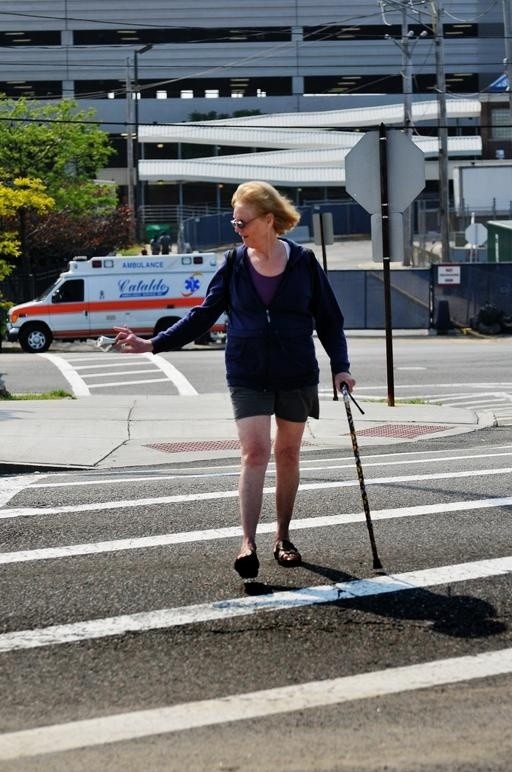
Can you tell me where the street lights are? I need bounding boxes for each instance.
[132,41,155,245]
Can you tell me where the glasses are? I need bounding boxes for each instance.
[230,215,260,229]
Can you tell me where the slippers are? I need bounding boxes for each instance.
[235,552,259,578]
[273,540,301,567]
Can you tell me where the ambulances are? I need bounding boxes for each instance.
[6,251,228,354]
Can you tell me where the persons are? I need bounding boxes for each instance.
[109,179,359,579]
[158,231,172,255]
[149,234,160,255]
